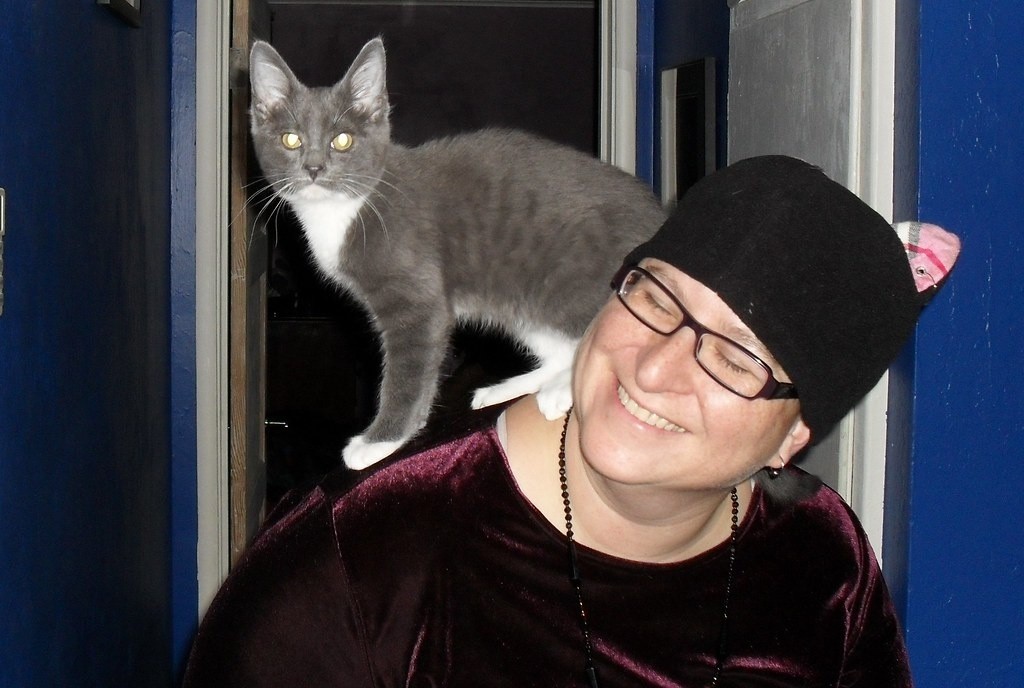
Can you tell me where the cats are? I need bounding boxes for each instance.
[249,37,667,470]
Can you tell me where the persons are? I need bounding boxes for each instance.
[178,153,922,688]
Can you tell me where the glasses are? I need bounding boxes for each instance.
[615,265,800,400]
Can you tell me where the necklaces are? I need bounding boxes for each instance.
[559,403,741,688]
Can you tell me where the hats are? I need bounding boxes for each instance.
[609,154,925,449]
